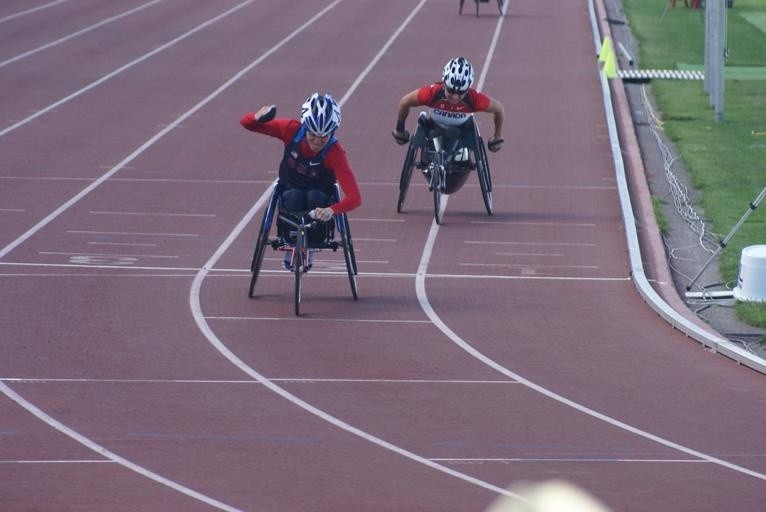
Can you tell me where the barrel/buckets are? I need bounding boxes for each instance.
[726,245,766,304]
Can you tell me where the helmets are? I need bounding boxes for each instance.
[300,91,343,137]
[441,56,475,93]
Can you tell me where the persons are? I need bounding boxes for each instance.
[393,57,505,194]
[240,92,362,272]
